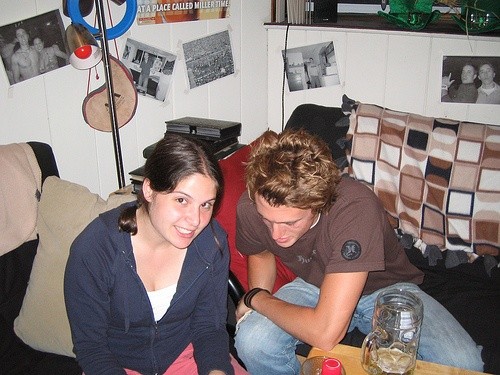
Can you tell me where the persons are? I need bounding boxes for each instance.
[307,58,321,88]
[64,133,251,375]
[2,27,69,83]
[441,61,500,105]
[234,129,484,375]
[124,46,152,92]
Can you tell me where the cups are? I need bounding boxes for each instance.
[360,289,424,375]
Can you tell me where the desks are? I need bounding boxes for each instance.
[301,343,495,375]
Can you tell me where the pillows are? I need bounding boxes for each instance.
[13,176,137,358]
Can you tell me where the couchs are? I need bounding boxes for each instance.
[211,94,500,375]
[0,142,59,375]
